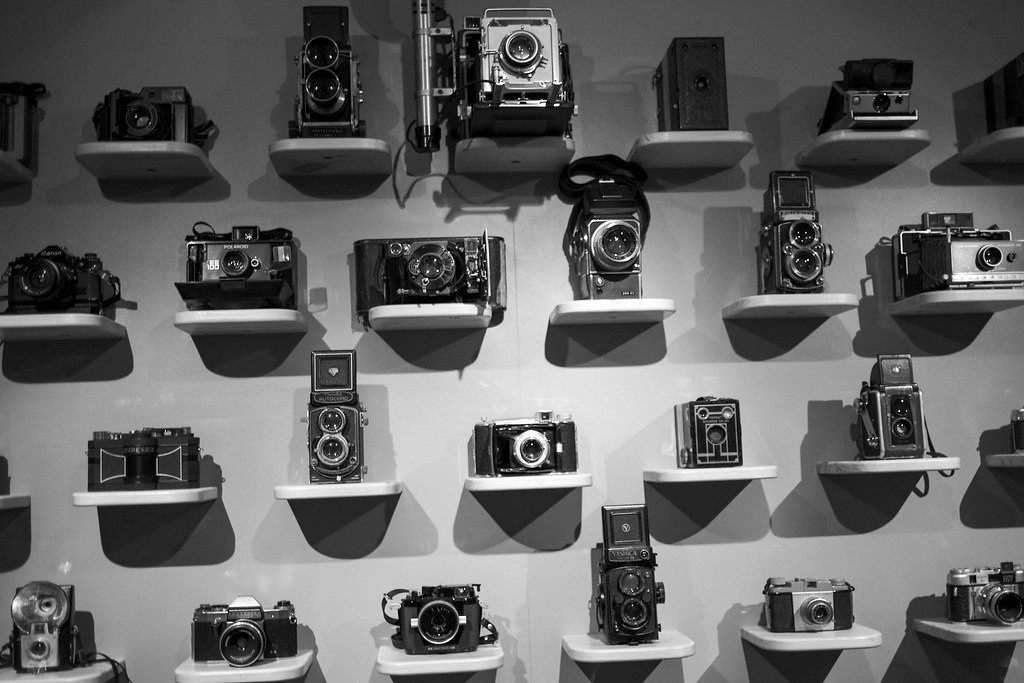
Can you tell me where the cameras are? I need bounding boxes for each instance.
[397,585,483,656]
[596,503,666,647]
[10,580,80,672]
[946,561,1024,625]
[0,0,1024,493]
[189,596,298,667]
[763,577,854,633]
